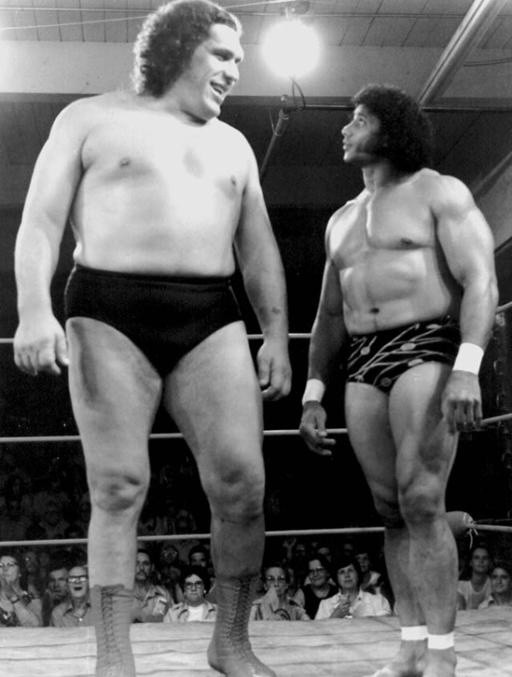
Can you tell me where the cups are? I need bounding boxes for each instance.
[338,593,353,617]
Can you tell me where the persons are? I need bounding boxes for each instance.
[12,1,293,677]
[298,83,500,677]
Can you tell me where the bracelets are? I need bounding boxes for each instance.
[452,341,484,376]
[300,378,328,406]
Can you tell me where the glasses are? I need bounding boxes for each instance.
[0,561,20,569]
[66,575,87,582]
[266,575,285,581]
[184,581,205,589]
